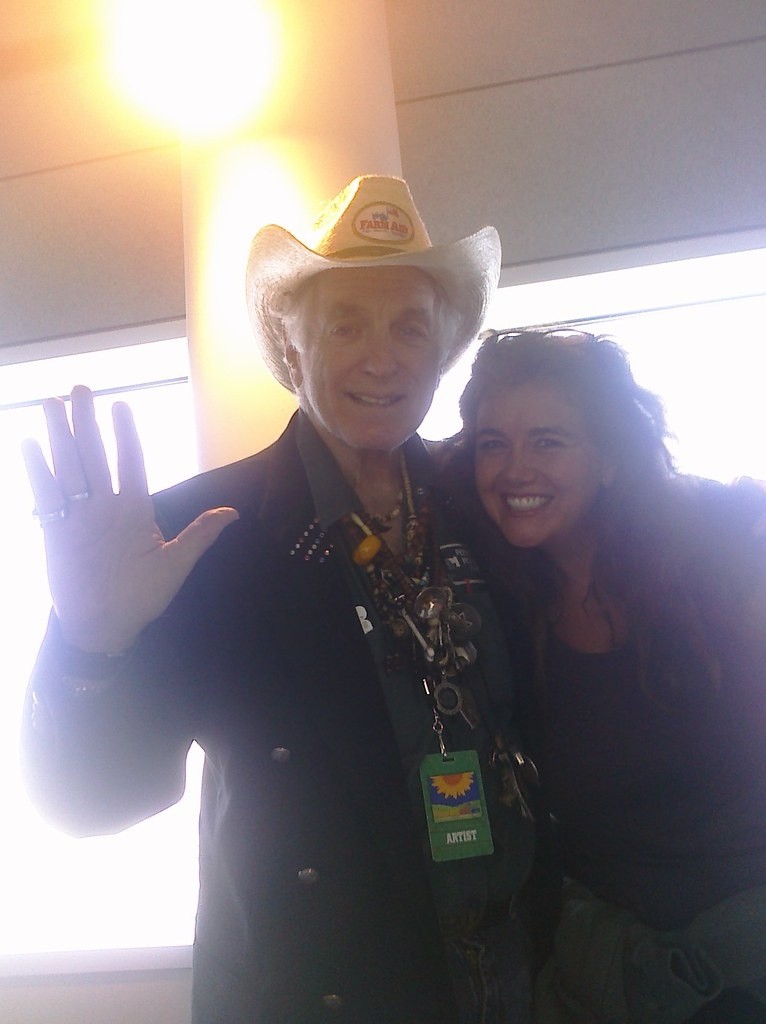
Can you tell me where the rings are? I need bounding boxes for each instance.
[63,490,89,503]
[33,508,68,526]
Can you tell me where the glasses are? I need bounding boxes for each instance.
[489,326,595,352]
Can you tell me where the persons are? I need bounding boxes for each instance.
[435,329,764,1024]
[22,176,536,1024]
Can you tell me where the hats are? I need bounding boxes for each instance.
[252,175,500,395]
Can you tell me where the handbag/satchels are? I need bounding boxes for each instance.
[533,872,766,1024]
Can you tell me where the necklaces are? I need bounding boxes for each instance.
[343,446,481,729]
